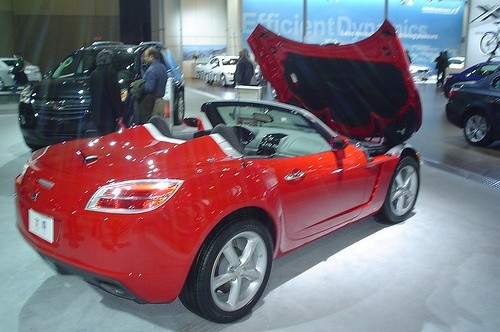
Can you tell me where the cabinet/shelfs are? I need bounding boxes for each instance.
[234,85,272,126]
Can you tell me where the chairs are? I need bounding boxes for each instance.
[210,123,244,155]
[147,115,173,138]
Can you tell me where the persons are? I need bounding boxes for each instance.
[228,48,255,120]
[89,49,123,136]
[9,52,29,93]
[130,46,168,126]
[434,51,451,85]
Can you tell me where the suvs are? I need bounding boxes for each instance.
[17,40,186,151]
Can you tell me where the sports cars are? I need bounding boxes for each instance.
[16,19,422,328]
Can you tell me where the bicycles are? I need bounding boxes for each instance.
[478,21,500,55]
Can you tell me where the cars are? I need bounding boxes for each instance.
[0,58,42,91]
[444,69,500,146]
[441,60,500,97]
[203,56,242,87]
